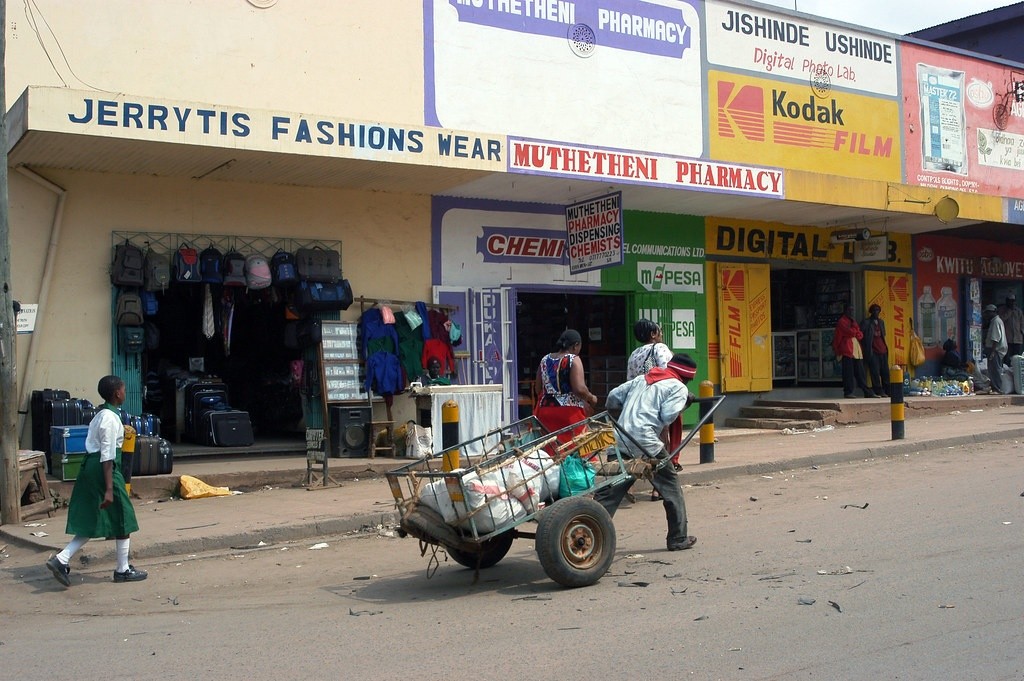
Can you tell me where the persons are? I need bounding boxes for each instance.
[46,376,148,586]
[537,327,598,463]
[605,353,697,550]
[997,294,1024,365]
[858,305,892,395]
[940,339,988,393]
[983,304,1009,395]
[834,303,880,397]
[628,319,683,502]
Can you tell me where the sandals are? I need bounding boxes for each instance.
[651,488,663,500]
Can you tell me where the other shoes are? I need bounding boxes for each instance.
[872,394,881,398]
[989,389,1000,395]
[674,464,683,472]
[668,536,697,551]
[844,394,856,398]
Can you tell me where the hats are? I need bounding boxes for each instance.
[427,357,441,368]
[983,304,996,313]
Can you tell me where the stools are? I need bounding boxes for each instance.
[366,420,397,457]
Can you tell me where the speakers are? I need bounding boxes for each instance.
[330,405,372,458]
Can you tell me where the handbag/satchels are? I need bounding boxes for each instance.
[296,276,354,310]
[405,424,432,458]
[643,343,657,374]
[559,451,597,498]
[296,246,340,282]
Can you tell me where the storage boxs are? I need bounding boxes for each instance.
[51,452,86,481]
[50,425,89,454]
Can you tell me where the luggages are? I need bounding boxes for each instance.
[183,378,256,445]
[31,389,172,482]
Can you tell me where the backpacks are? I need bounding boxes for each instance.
[112,238,298,353]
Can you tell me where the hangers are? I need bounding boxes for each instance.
[434,331,440,340]
[429,304,438,312]
[377,340,387,353]
[370,299,380,309]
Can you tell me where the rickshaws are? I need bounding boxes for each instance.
[386,394,727,586]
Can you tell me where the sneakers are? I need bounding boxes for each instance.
[114,565,147,582]
[46,555,71,587]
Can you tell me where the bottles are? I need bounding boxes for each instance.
[936,286,958,351]
[903,372,974,397]
[917,286,937,349]
